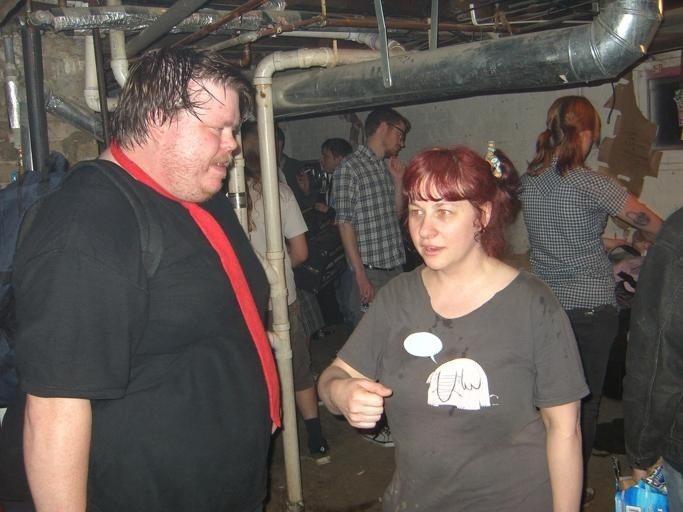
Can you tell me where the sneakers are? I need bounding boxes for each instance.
[582,488,594,506]
[359,427,395,448]
[307,447,331,465]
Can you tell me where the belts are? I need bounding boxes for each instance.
[364,264,391,270]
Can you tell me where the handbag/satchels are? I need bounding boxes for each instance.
[309,323,355,381]
[287,203,346,295]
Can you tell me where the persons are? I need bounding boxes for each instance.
[316,145,589,511]
[225,106,413,464]
[621,206,683,511]
[516,95,665,505]
[13,47,283,512]
